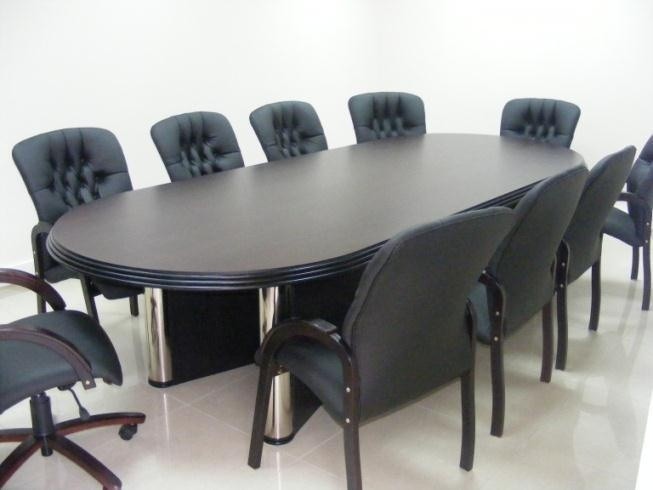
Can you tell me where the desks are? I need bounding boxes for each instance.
[44,133,584,387]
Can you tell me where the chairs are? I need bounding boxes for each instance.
[11,127,149,326]
[501,99,580,148]
[250,100,327,161]
[247,207,517,488]
[150,112,245,182]
[562,144,637,370]
[607,134,652,312]
[0,267,146,489]
[474,165,590,436]
[348,91,427,143]
